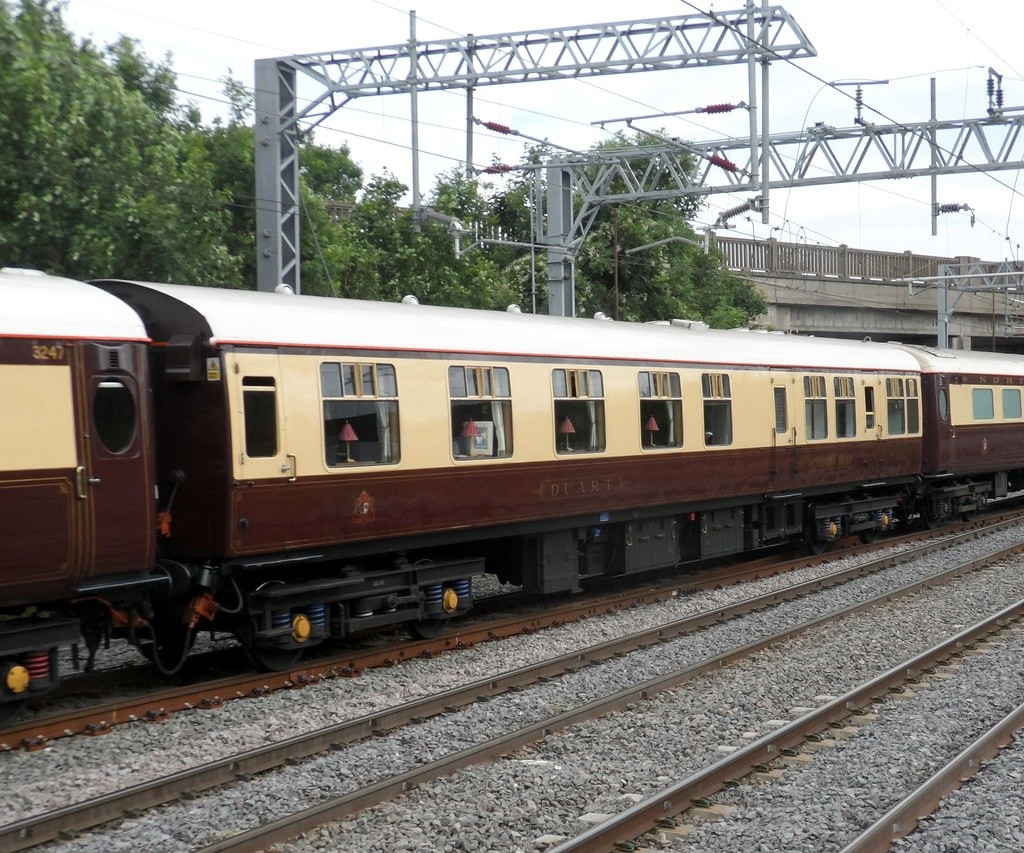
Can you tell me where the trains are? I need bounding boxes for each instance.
[1,261,1024,717]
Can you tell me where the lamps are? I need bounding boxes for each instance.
[642,414,661,446]
[338,419,358,464]
[559,415,576,453]
[461,418,480,458]
